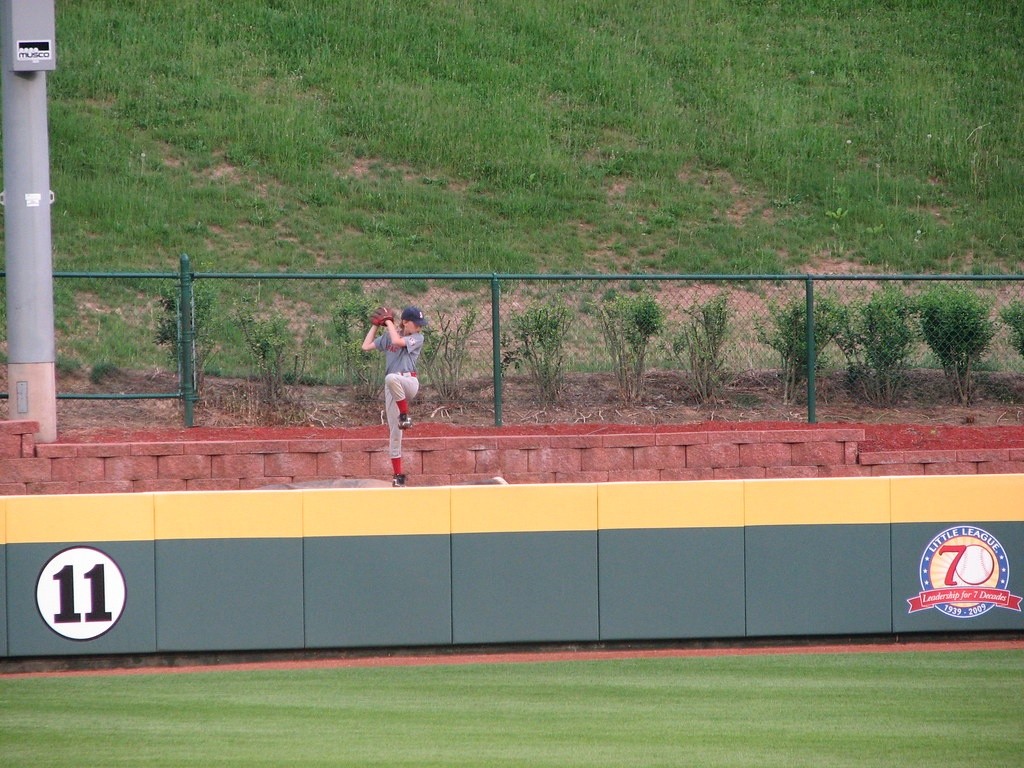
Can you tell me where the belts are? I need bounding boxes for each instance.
[401,372,416,377]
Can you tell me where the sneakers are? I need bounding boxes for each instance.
[398,413,412,429]
[392,473,406,487]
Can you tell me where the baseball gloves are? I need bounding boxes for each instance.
[369,306,395,326]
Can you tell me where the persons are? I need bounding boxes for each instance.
[361,306,429,488]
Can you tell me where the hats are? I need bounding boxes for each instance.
[401,306,427,326]
